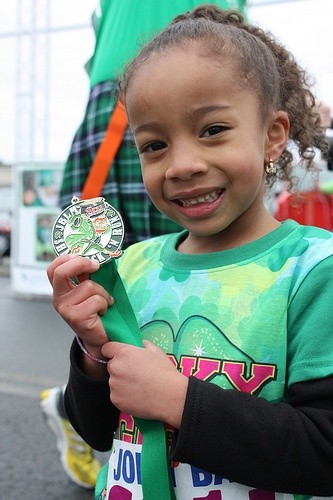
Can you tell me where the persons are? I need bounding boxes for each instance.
[46,6,333,500]
[37,0,248,489]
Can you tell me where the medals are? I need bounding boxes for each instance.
[51,194,125,267]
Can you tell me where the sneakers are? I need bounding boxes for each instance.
[37,382,101,490]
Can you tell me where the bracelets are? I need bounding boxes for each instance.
[75,332,109,367]
